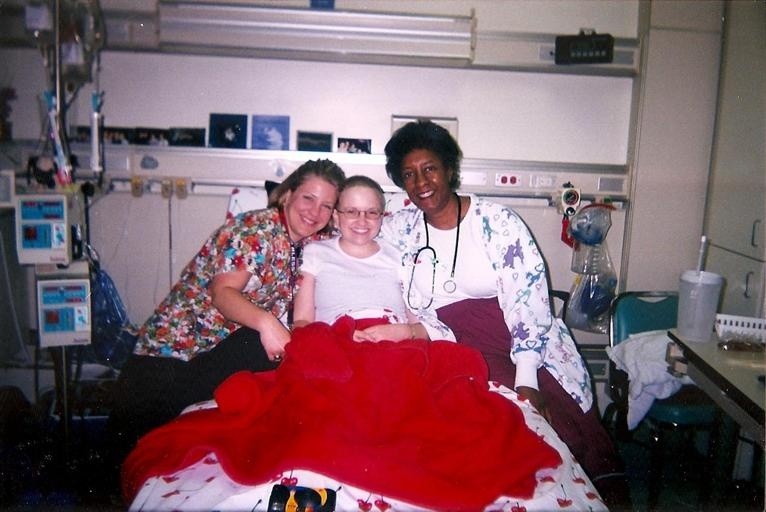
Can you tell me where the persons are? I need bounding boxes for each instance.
[378,119,595,416]
[116,158,347,429]
[292,176,456,343]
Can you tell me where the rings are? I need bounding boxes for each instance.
[275,356,280,359]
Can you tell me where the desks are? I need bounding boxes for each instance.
[665,324,765,509]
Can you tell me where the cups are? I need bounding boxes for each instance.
[678,268,724,344]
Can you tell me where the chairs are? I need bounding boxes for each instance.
[603,291,716,454]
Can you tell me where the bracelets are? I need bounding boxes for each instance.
[409,323,416,340]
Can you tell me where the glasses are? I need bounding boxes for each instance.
[336,206,382,220]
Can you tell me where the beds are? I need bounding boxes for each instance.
[134,186,609,512]
